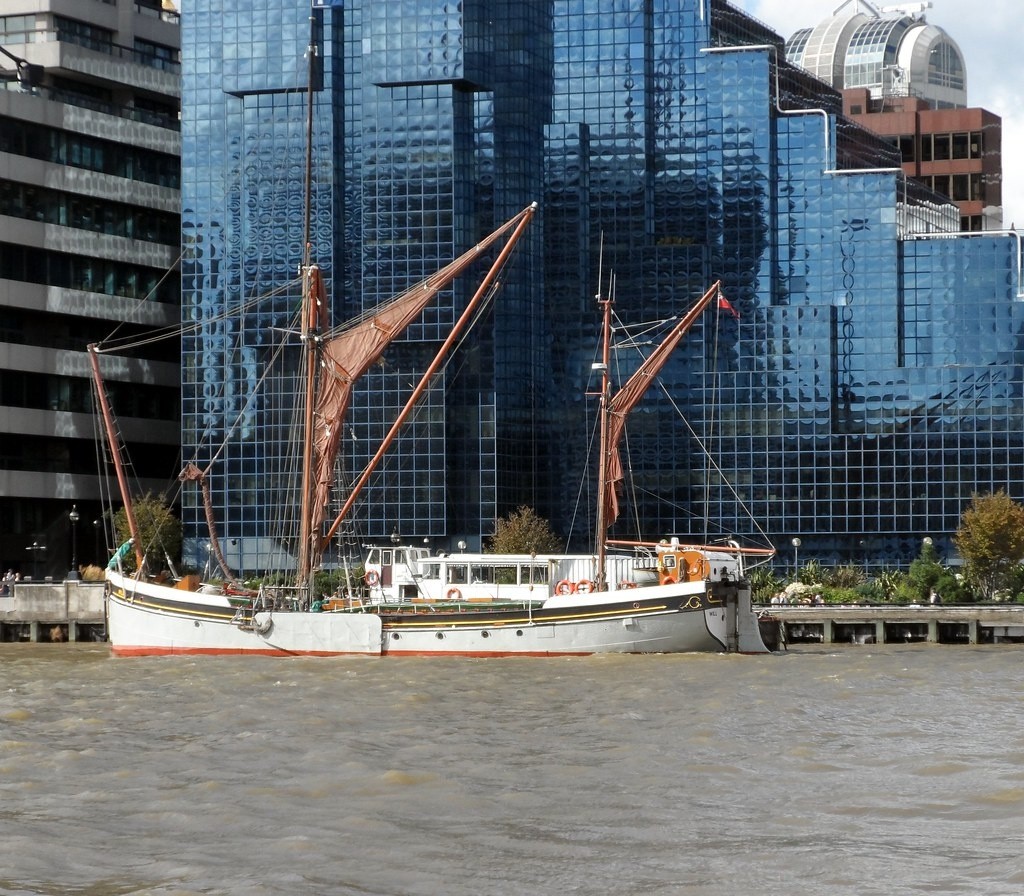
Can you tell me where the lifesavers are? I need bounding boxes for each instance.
[662,576,676,585]
[555,579,574,596]
[618,580,636,589]
[364,569,379,586]
[574,579,594,594]
[447,588,461,599]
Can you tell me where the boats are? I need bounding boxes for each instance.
[85,13,776,658]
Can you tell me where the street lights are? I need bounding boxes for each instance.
[793,538,801,604]
[26,542,48,580]
[67,505,79,579]
[458,541,466,554]
[92,520,101,567]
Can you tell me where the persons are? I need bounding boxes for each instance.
[770,590,825,606]
[0,568,22,597]
[929,586,944,607]
